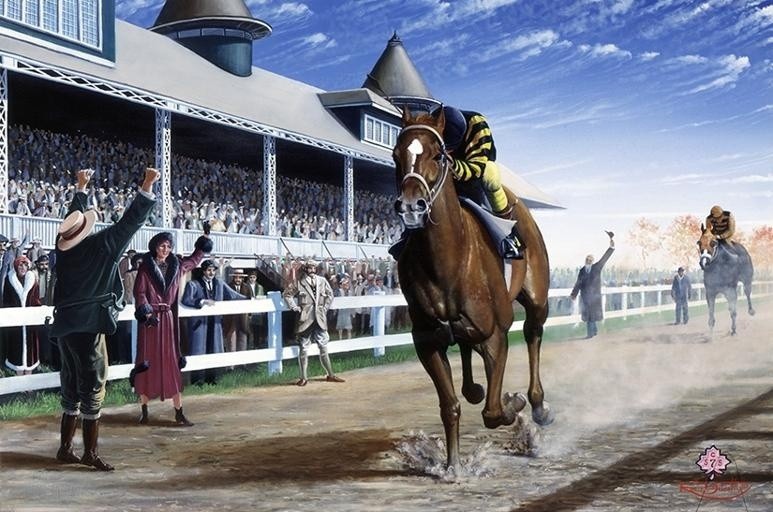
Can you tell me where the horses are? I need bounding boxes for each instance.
[696,222,755,338]
[391,102,550,479]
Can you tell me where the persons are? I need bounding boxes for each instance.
[670,269,694,324]
[569,232,615,336]
[706,206,737,250]
[435,107,521,256]
[0,124,405,470]
